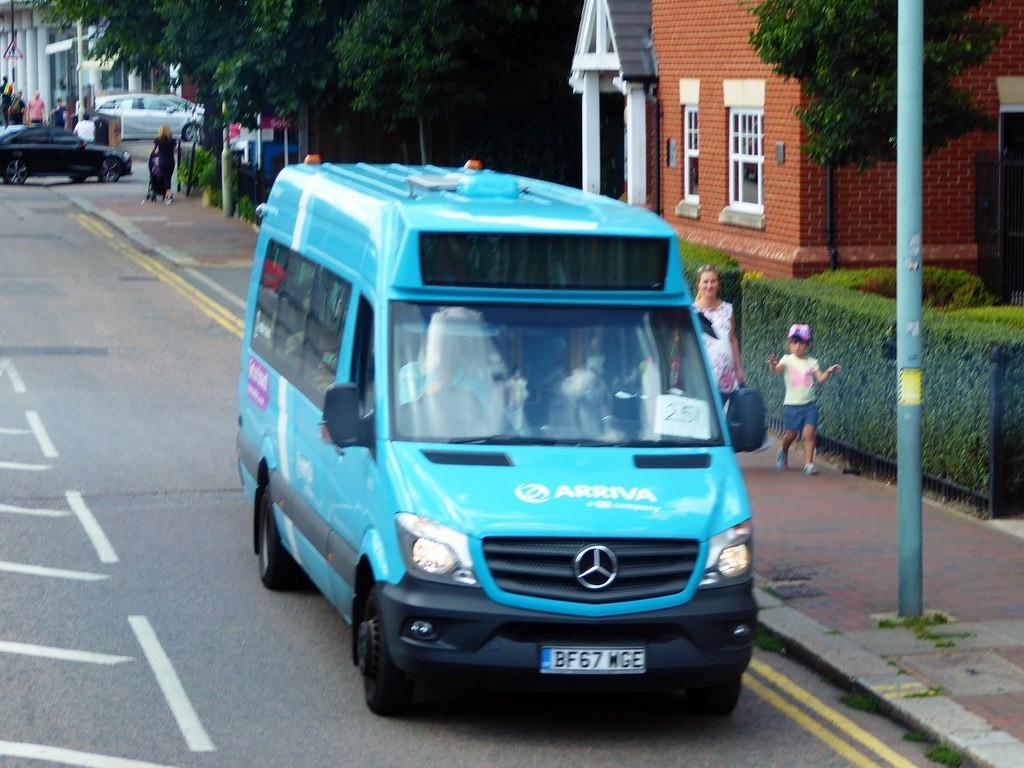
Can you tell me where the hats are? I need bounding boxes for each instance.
[787,323,812,343]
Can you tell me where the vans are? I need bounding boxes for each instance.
[238,154,764,717]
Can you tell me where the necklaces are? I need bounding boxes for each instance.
[705,298,717,308]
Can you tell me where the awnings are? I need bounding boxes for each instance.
[46,33,105,55]
[83,52,120,71]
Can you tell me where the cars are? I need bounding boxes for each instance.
[0,125,134,182]
[95,94,205,142]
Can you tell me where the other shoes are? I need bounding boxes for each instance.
[802,463,820,475]
[165,196,173,206]
[776,449,788,471]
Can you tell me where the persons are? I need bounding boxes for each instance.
[691,264,745,405]
[400,333,526,435]
[25,92,47,124]
[229,122,241,138]
[50,99,67,128]
[73,113,95,144]
[150,125,177,205]
[0,77,12,125]
[8,92,25,125]
[767,324,842,475]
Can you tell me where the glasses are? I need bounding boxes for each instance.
[697,265,715,273]
